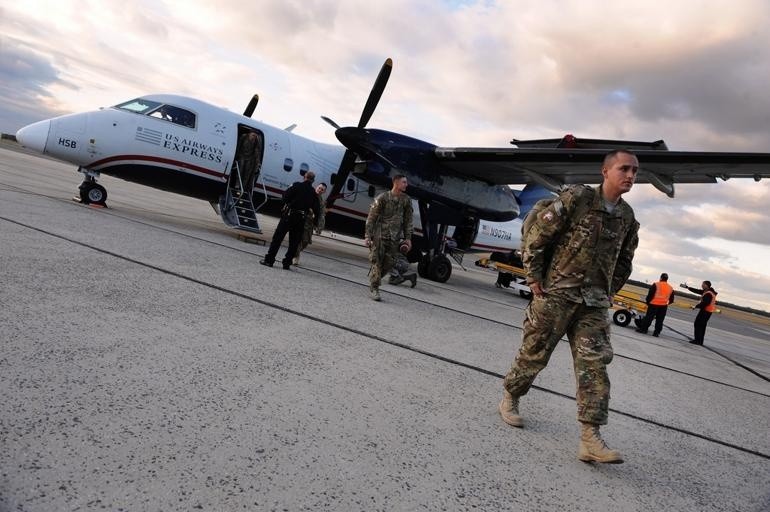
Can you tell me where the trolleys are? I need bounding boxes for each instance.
[471,252,648,330]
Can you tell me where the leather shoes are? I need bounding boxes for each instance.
[262,257,275,266]
[282,260,294,269]
[651,334,659,337]
[690,339,701,343]
[636,329,647,334]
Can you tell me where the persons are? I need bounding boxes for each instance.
[388,226,417,288]
[635,273,674,337]
[292,182,328,265]
[235,129,262,188]
[259,171,321,270]
[499,149,640,464]
[680,280,721,346]
[364,173,413,299]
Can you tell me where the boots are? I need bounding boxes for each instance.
[368,285,383,302]
[410,272,417,288]
[292,255,299,265]
[497,391,523,428]
[578,420,623,463]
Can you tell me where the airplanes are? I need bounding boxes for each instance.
[14,57,770,283]
